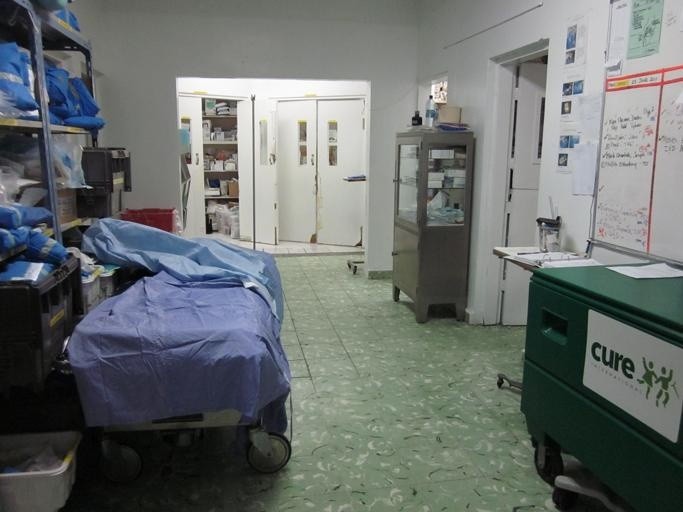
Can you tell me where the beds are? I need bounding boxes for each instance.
[48,235,293,474]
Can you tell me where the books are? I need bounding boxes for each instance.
[498,246,603,270]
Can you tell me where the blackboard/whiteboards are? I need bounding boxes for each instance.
[588,64,683,267]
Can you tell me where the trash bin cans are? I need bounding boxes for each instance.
[121,207,178,234]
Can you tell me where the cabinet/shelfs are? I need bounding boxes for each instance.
[179,94,253,242]
[0,1,99,267]
[392,131,474,324]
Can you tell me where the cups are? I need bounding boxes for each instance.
[537,220,561,251]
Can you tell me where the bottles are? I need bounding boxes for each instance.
[453,203,459,222]
[411,111,421,126]
[424,95,435,127]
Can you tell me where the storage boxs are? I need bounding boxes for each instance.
[54,130,91,191]
[56,188,79,224]
[1,432,85,512]
[2,251,120,399]
